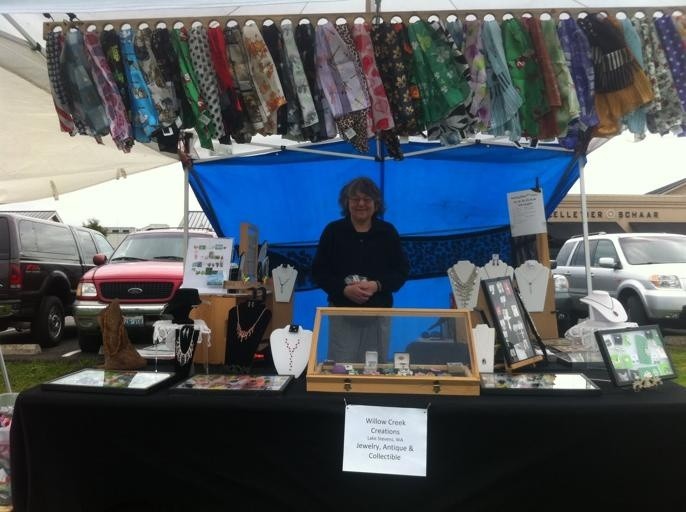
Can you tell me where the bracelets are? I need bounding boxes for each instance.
[376,280,381,293]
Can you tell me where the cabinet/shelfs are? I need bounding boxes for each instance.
[186,293,300,367]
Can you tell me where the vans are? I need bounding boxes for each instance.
[1,213,115,347]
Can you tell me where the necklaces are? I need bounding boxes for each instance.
[284,336,300,372]
[484,262,509,281]
[231,305,269,342]
[102,306,126,357]
[175,326,195,365]
[450,266,479,309]
[519,269,544,295]
[276,272,293,296]
[583,296,620,318]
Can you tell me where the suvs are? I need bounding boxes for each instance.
[75,230,216,352]
[557,233,686,327]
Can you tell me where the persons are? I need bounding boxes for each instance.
[311,175,411,363]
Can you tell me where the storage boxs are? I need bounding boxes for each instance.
[304,305,482,395]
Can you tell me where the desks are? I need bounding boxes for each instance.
[9,370,686,511]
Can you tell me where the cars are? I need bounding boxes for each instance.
[550,258,569,335]
